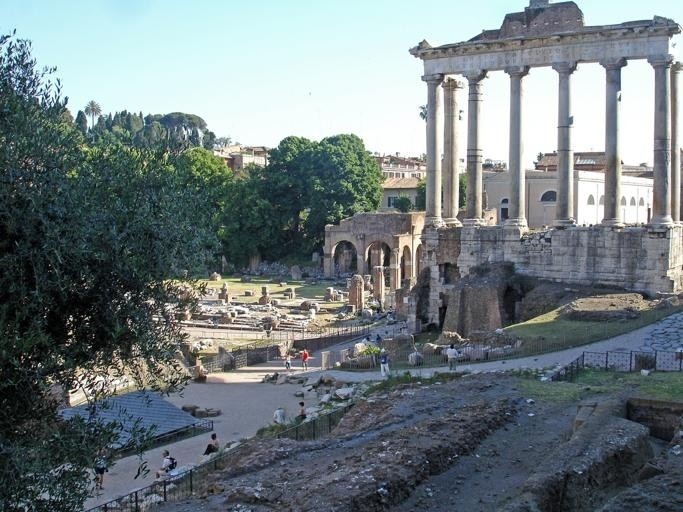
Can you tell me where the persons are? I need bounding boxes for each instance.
[201,432,220,456]
[301,348,309,371]
[446,342,458,371]
[93,444,106,491]
[378,349,391,377]
[154,449,172,479]
[271,406,285,426]
[282,351,296,371]
[360,330,390,346]
[295,401,307,422]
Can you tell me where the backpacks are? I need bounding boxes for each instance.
[167,457,177,469]
